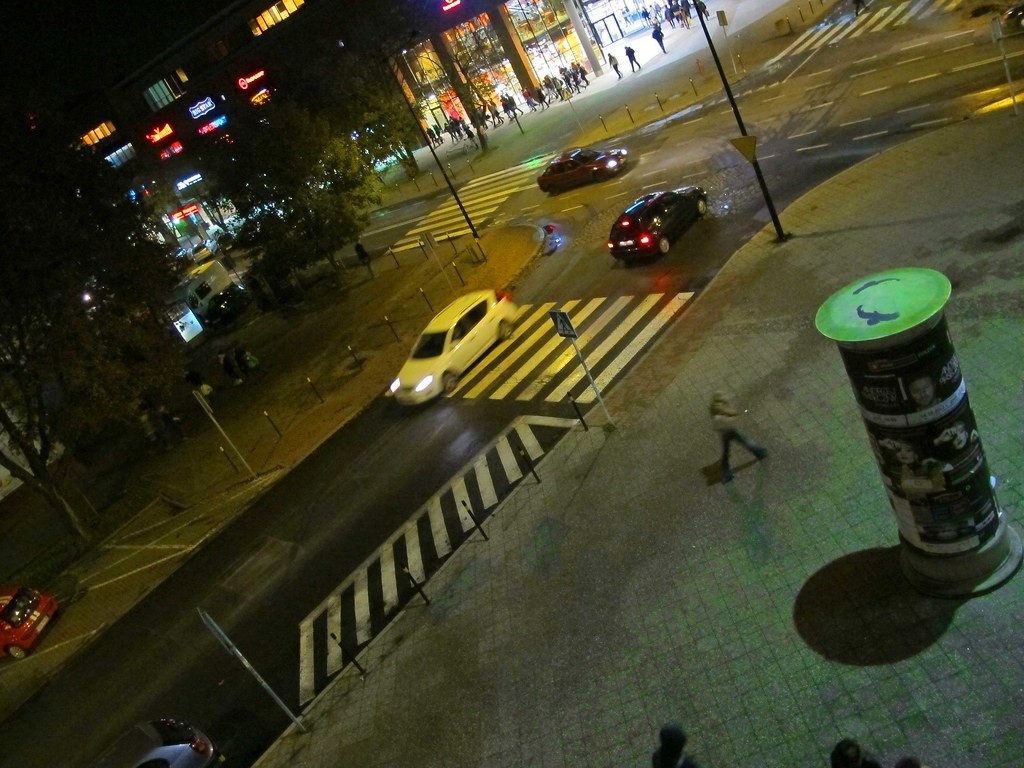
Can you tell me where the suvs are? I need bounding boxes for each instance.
[609,184,709,264]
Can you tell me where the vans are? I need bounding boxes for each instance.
[171,260,233,328]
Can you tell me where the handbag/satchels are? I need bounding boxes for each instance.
[712,415,737,433]
[494,109,500,117]
[583,68,589,75]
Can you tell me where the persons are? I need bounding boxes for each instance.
[651,721,699,768]
[707,390,768,485]
[640,0,709,30]
[652,27,666,54]
[607,53,623,80]
[624,47,641,72]
[830,737,921,767]
[426,61,590,149]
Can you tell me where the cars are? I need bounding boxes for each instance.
[386,287,515,406]
[1,583,58,661]
[538,143,628,196]
[194,237,221,265]
[173,246,194,262]
[208,282,252,322]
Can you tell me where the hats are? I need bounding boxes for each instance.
[713,390,725,401]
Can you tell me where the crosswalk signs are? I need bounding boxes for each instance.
[547,311,577,340]
